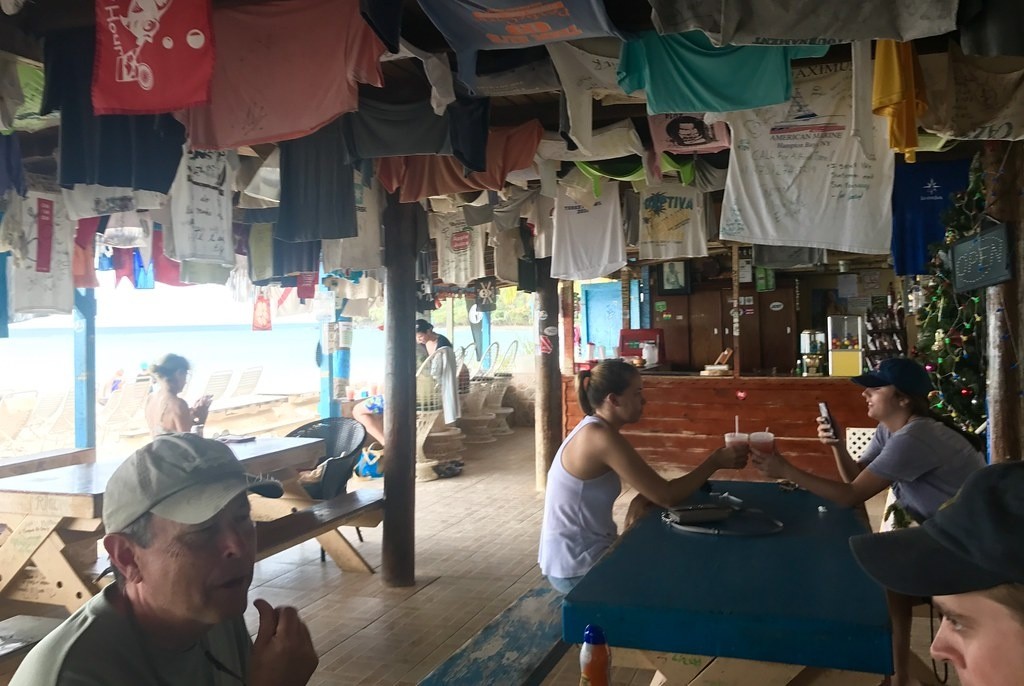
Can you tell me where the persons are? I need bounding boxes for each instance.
[749,357,988,686]
[144,355,213,437]
[415,318,453,361]
[537,359,751,597]
[849,460,1024,686]
[9,431,320,686]
[352,367,385,447]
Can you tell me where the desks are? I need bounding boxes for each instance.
[562,480,894,685]
[0,435,375,615]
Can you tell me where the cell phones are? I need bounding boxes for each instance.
[818,400,838,439]
[204,393,214,399]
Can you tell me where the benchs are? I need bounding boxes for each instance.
[255,489,382,563]
[416,576,572,686]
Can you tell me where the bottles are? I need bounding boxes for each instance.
[864,281,906,372]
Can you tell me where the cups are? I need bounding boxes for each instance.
[750,431,775,453]
[723,432,749,447]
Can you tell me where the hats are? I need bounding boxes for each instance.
[850,461,1024,596]
[102,432,284,532]
[850,358,933,396]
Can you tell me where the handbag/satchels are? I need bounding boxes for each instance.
[352,442,384,481]
[662,492,783,535]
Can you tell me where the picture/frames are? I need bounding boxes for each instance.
[656,260,692,295]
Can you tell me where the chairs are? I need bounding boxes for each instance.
[204,368,232,399]
[0,390,34,454]
[365,339,519,482]
[107,375,153,442]
[46,377,74,449]
[235,368,262,398]
[283,417,365,563]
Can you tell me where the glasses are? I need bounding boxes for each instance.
[416,324,421,329]
